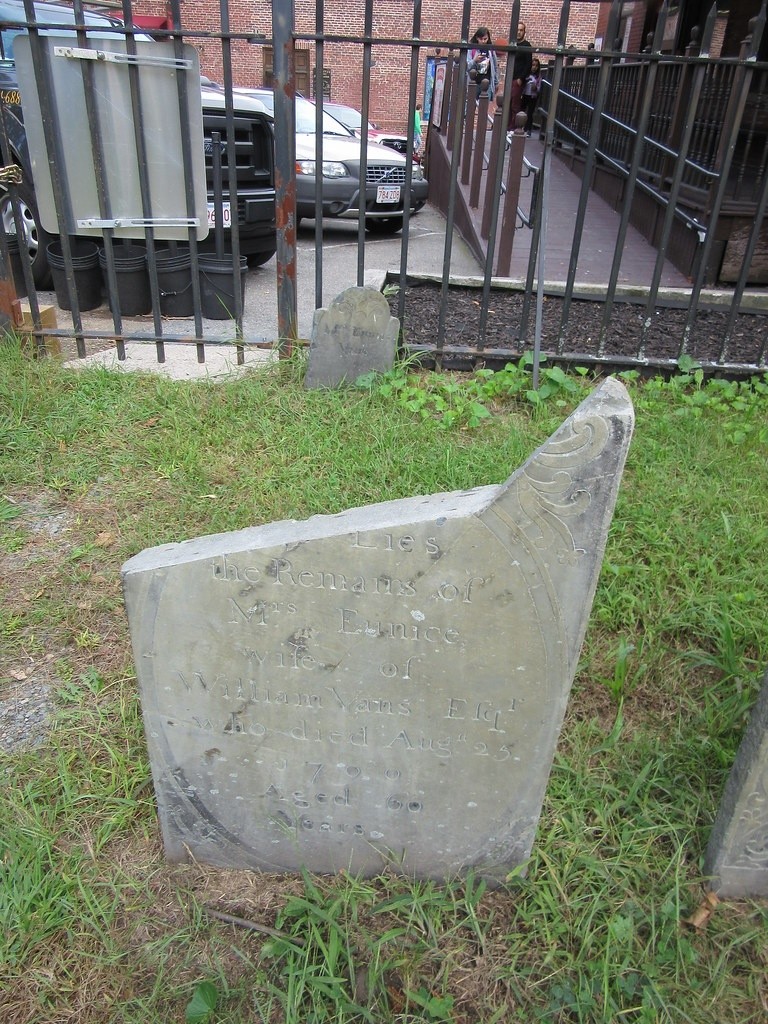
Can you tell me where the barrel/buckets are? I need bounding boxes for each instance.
[145,247,195,316]
[46,239,102,311]
[5,233,29,299]
[198,253,248,320]
[99,244,152,317]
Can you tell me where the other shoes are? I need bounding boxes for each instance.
[526,130,531,136]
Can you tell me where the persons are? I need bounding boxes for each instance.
[504,21,542,138]
[467,27,500,103]
[413,104,424,154]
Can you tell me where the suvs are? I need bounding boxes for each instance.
[217,85,430,237]
[1,0,279,293]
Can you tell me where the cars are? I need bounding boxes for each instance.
[306,97,420,167]
